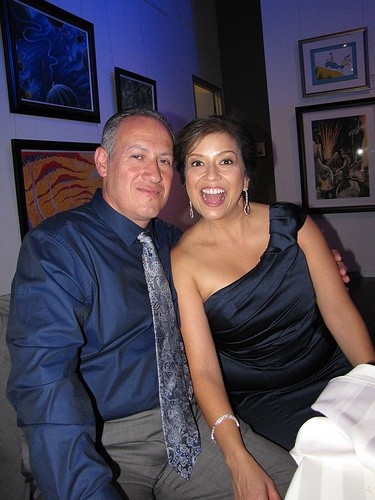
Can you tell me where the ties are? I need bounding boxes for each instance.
[138,231,203,479]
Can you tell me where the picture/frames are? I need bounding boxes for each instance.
[0,0,101,123]
[297,26,371,98]
[295,97,375,214]
[11,138,102,243]
[114,66,158,116]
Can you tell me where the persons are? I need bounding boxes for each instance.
[169,114,375,500]
[5,107,350,499]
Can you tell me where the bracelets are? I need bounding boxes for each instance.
[210,414,240,444]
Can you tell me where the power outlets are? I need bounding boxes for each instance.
[255,142,265,156]
